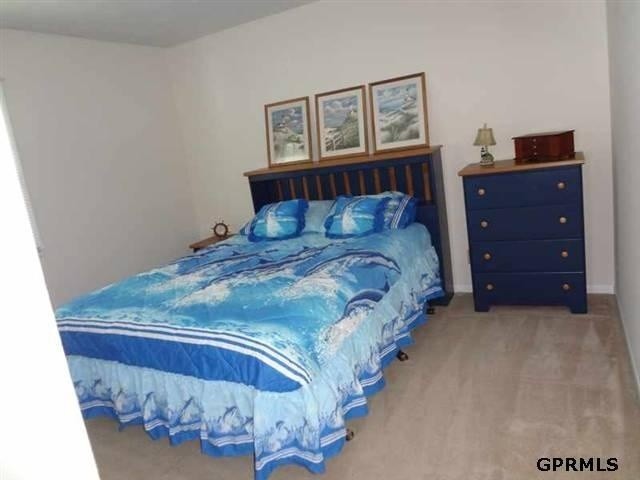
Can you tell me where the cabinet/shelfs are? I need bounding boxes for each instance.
[457,151,587,315]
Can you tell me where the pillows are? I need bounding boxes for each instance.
[321,189,420,239]
[240,199,335,243]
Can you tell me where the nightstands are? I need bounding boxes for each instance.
[188,235,229,252]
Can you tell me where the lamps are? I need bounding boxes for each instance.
[472,123,498,166]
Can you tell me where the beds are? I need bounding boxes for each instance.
[54,145,454,480]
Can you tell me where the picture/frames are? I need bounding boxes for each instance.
[368,72,429,155]
[264,95,312,169]
[314,85,369,162]
